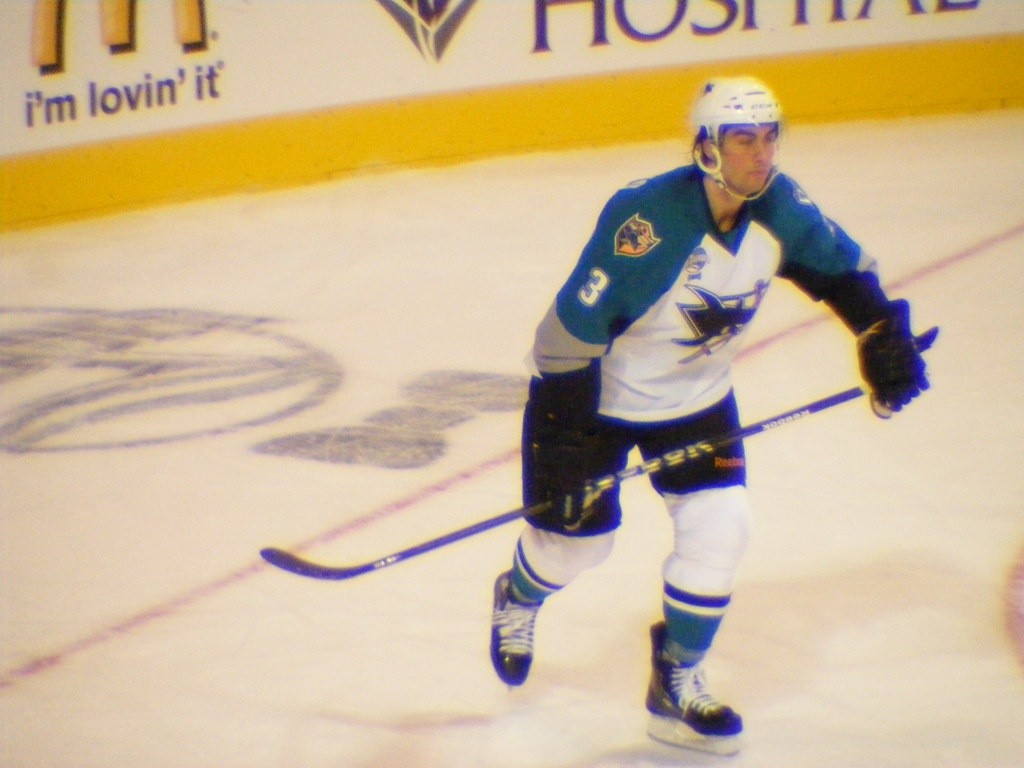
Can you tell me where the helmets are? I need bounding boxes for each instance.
[690,78,783,141]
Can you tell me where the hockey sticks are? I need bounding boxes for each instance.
[256,323,942,584]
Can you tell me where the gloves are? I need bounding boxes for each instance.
[530,417,600,534]
[858,300,932,418]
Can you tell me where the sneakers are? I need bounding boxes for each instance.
[644,622,745,758]
[490,573,539,687]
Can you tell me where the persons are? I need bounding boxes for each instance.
[490,79,932,736]
